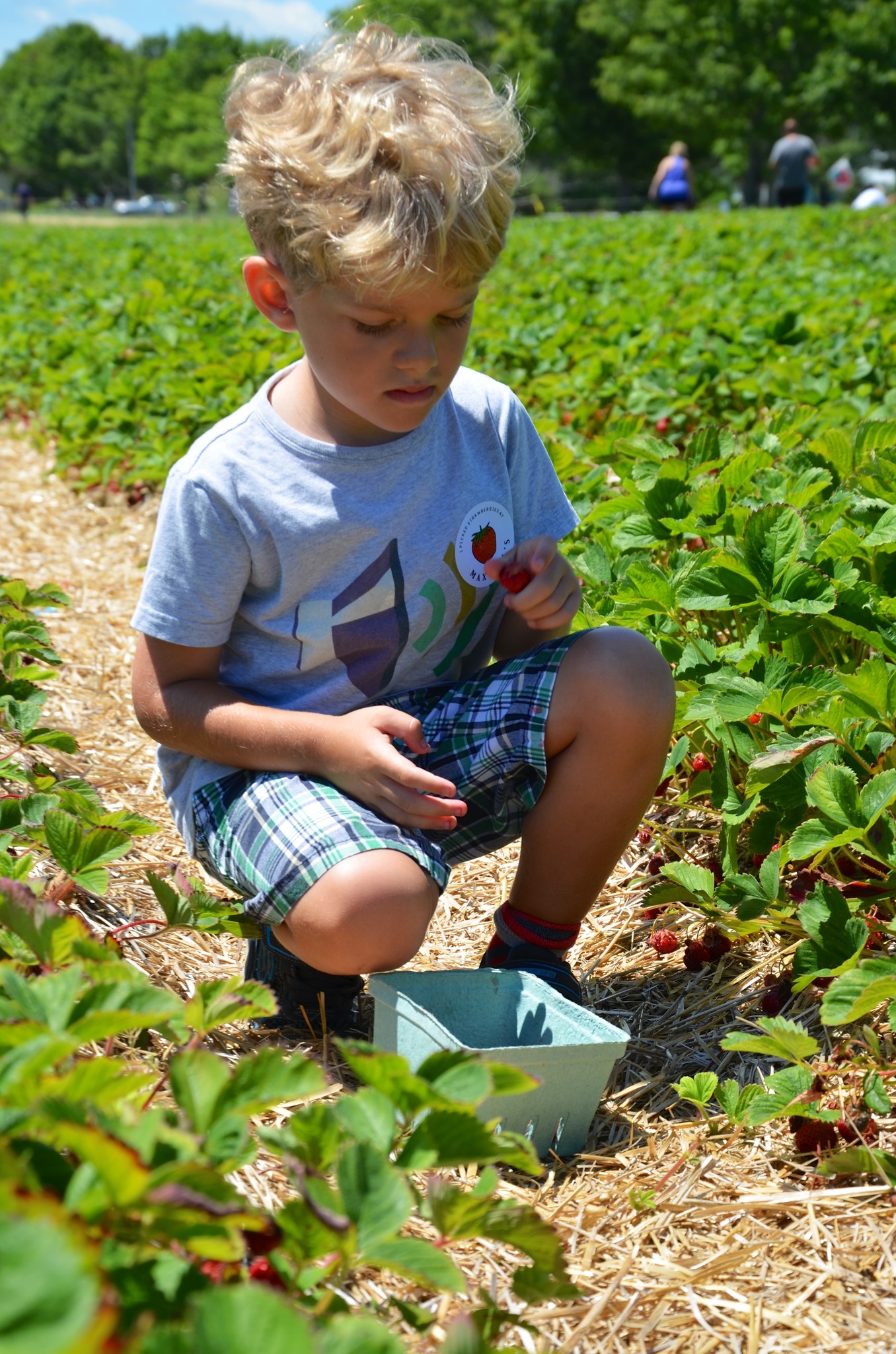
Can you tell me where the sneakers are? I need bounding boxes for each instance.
[242,921,364,1043]
[477,939,582,1010]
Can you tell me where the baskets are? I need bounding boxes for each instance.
[366,968,631,1161]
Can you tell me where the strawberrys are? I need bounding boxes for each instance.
[191,1225,290,1296]
[110,457,150,506]
[501,405,885,1154]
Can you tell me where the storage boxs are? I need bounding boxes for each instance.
[368,969,631,1154]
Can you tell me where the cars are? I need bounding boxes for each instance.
[116,192,179,216]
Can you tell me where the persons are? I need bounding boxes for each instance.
[644,118,896,212]
[127,20,677,1042]
